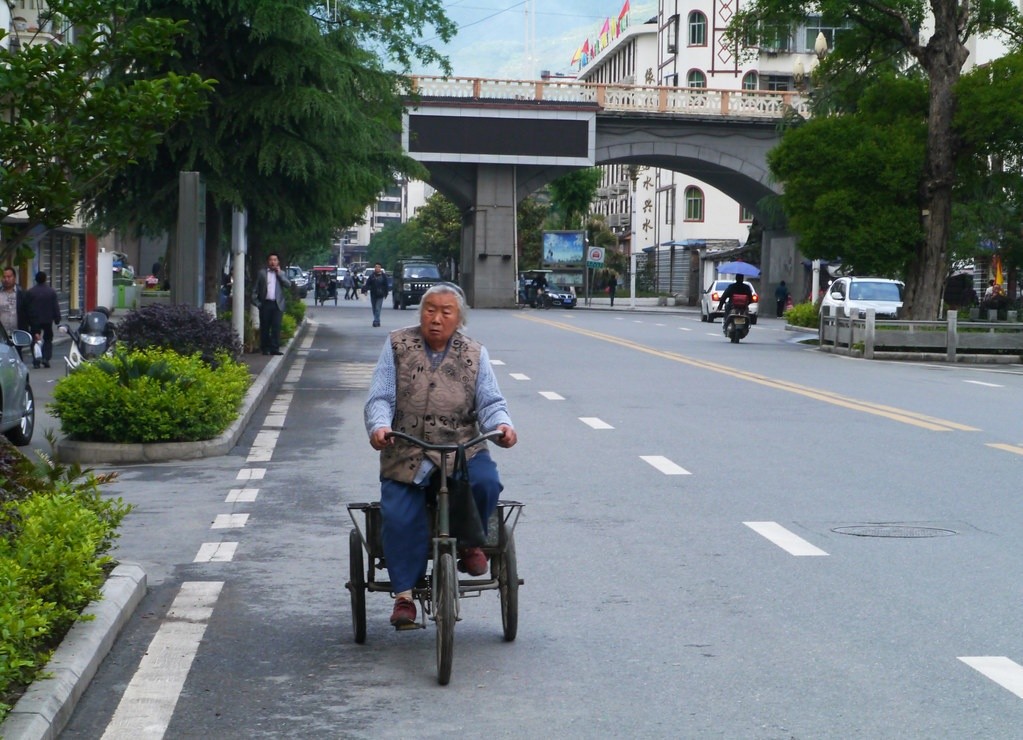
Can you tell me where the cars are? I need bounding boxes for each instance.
[0,321,36,446]
[819,276,905,319]
[699,279,759,324]
[518,280,577,309]
[285,262,393,298]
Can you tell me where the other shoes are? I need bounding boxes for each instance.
[263,350,269,355]
[270,351,283,355]
[373,320,380,328]
[34,364,40,369]
[42,361,49,368]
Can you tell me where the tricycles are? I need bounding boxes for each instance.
[345,431,525,684]
[312,265,338,305]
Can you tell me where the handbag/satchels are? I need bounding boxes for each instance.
[33,340,41,359]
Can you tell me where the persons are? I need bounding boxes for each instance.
[250,254,291,356]
[152,257,164,276]
[531,272,549,307]
[0,266,61,369]
[984,279,994,297]
[607,274,617,306]
[363,279,518,626]
[362,264,389,327]
[715,273,753,332]
[774,280,788,319]
[343,270,375,300]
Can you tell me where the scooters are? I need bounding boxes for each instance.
[57,306,118,377]
[719,294,752,343]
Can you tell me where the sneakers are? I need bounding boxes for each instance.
[456,551,487,576]
[390,597,416,626]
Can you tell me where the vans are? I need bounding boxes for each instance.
[392,255,442,309]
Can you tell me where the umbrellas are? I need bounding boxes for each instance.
[715,261,762,277]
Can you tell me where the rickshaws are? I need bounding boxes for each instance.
[519,269,552,310]
